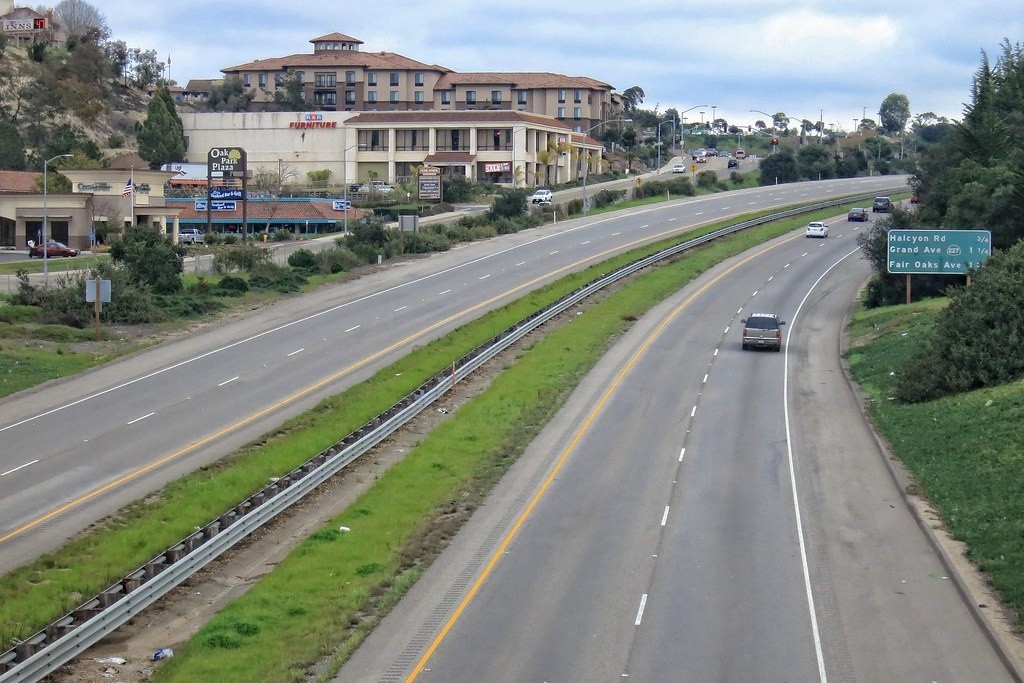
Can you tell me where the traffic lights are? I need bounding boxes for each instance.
[771,138,779,145]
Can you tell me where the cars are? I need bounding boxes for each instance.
[847,207,868,222]
[531,189,552,204]
[911,194,921,204]
[32,238,81,259]
[805,221,829,239]
[672,164,685,174]
[349,180,396,200]
[692,147,746,169]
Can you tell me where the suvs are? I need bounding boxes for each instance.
[872,196,895,213]
[741,312,786,352]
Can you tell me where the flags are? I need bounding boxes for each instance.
[122,178,136,198]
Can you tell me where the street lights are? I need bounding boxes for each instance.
[863,106,867,119]
[789,116,806,147]
[583,119,632,217]
[681,105,709,157]
[853,119,859,133]
[512,126,535,192]
[278,158,284,197]
[820,109,823,148]
[343,143,367,243]
[750,109,775,156]
[657,119,675,176]
[42,154,75,287]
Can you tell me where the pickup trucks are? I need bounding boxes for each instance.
[178,228,206,246]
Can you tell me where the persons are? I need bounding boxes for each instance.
[28,238,35,258]
